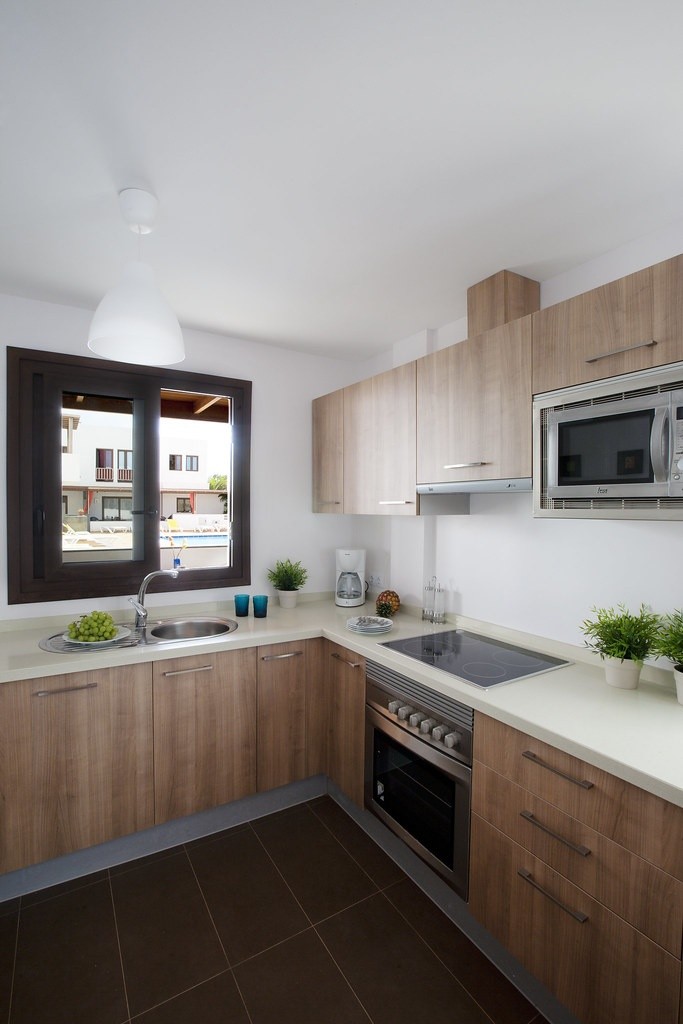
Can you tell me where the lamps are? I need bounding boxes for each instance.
[87,189,185,367]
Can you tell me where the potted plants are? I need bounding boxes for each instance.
[656,608,683,707]
[267,558,308,608]
[579,601,658,689]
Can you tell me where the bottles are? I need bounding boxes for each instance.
[422,581,434,621]
[433,584,444,623]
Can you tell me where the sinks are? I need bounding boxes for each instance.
[151,620,229,639]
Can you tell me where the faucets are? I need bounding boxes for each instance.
[127,569,178,627]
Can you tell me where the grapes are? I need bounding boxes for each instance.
[68,611,117,642]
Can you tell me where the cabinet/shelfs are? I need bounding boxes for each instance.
[311,387,344,514]
[470,707,683,1024]
[344,340,470,516]
[154,646,257,827]
[532,252,683,396]
[1,661,154,876]
[416,269,541,485]
[257,637,326,793]
[325,638,366,813]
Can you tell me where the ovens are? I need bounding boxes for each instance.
[364,659,474,903]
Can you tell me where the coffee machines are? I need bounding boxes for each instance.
[334,546,367,607]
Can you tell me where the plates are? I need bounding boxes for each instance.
[61,625,132,645]
[346,616,393,634]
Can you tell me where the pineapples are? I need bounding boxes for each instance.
[376,590,400,617]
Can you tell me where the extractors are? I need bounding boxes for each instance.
[416,478,532,495]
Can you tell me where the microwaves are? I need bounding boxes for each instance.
[531,359,683,523]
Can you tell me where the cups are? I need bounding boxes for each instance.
[234,594,249,617]
[253,595,268,618]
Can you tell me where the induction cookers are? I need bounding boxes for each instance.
[376,628,574,690]
